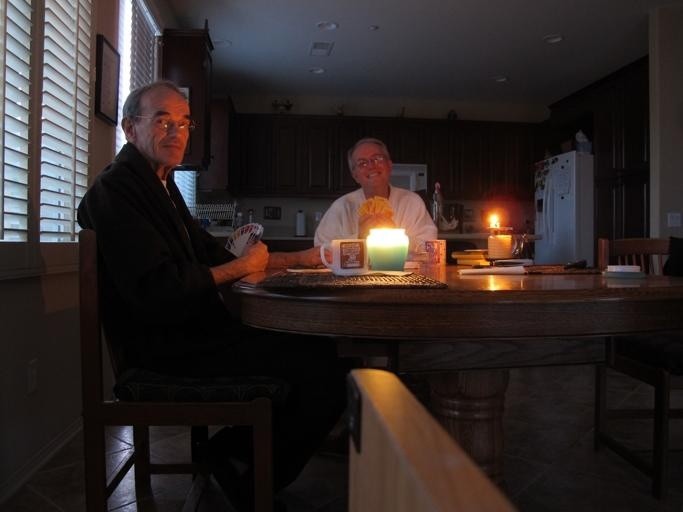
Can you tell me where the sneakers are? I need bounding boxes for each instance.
[197,426,254,512]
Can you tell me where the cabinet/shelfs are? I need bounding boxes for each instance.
[163,29,236,203]
[548,54,650,237]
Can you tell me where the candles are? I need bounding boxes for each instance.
[484,209,512,260]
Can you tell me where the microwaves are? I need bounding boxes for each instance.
[388,163,428,192]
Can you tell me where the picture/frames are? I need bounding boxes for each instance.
[93,34,120,128]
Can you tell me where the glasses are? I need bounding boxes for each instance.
[126,113,195,134]
[350,154,388,172]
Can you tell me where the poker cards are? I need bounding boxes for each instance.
[355,195,395,221]
[225,224,264,256]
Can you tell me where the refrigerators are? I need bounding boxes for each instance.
[531,150,596,267]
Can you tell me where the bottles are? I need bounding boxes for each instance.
[235,211,244,228]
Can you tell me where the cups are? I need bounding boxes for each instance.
[414,238,447,267]
[485,225,518,261]
[320,239,369,278]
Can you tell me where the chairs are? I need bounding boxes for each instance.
[593,236,682,497]
[79,227,274,511]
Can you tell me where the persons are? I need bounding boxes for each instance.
[312,138,438,454]
[77,79,347,511]
